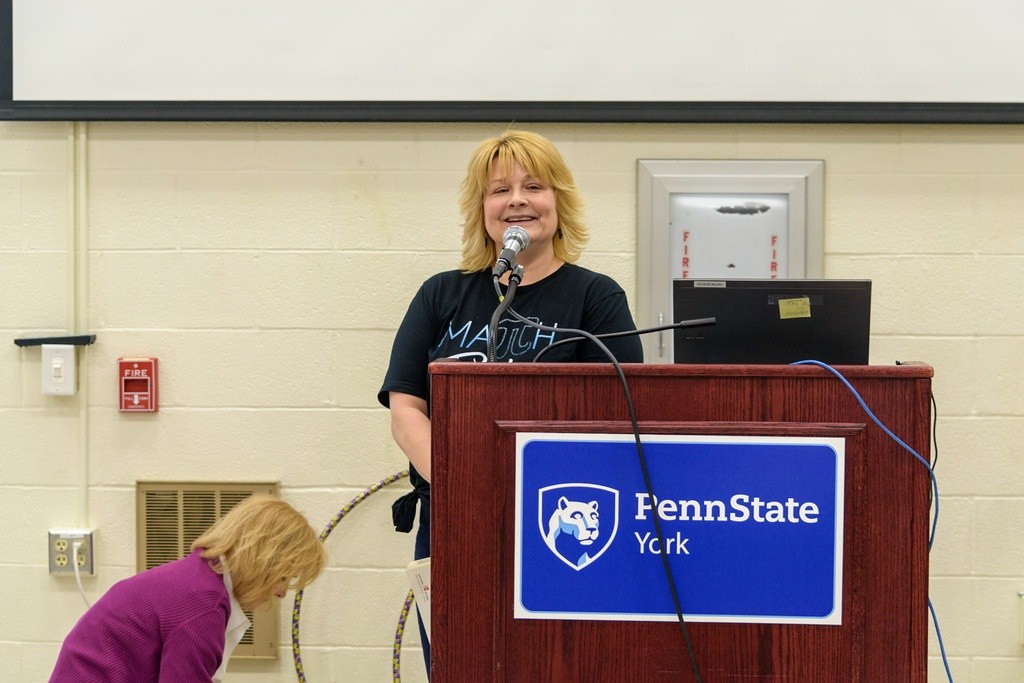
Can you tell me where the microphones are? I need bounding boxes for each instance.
[532,318,717,362]
[492,225,530,278]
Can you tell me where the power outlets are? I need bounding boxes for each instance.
[48,529,94,576]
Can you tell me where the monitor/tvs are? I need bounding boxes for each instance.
[673,278,872,366]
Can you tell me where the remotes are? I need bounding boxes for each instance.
[14,335,97,349]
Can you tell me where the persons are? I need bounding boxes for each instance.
[378,126,646,683]
[48,489,326,682]
